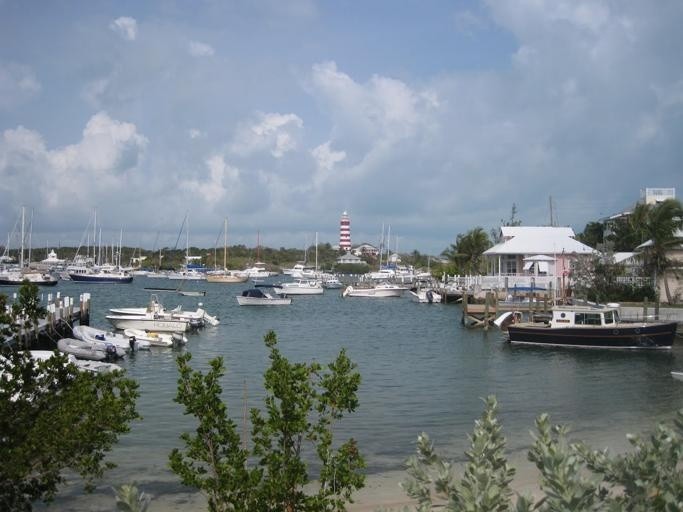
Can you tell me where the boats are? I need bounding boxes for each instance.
[235,284,292,306]
[272,279,324,295]
[410,288,442,304]
[340,281,402,299]
[0,293,221,403]
[508,303,678,351]
